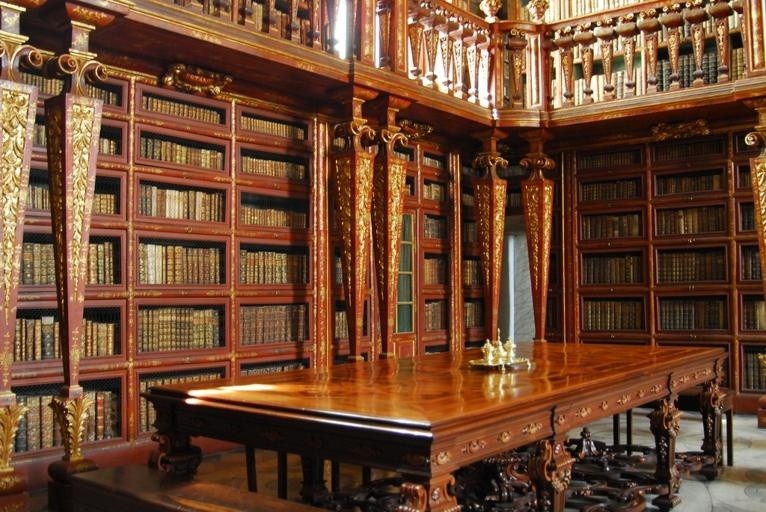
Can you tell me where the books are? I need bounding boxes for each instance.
[424,210,483,354]
[448,1,481,16]
[333,216,414,341]
[550,0,744,106]
[463,163,563,209]
[744,352,764,392]
[502,31,527,109]
[577,127,765,331]
[519,1,532,25]
[333,137,447,201]
[17,70,310,455]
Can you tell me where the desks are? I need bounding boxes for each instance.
[138,345,732,512]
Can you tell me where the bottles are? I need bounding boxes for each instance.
[492,327,507,365]
[506,371,520,398]
[479,338,496,365]
[480,373,495,401]
[502,336,516,364]
[493,366,507,400]
